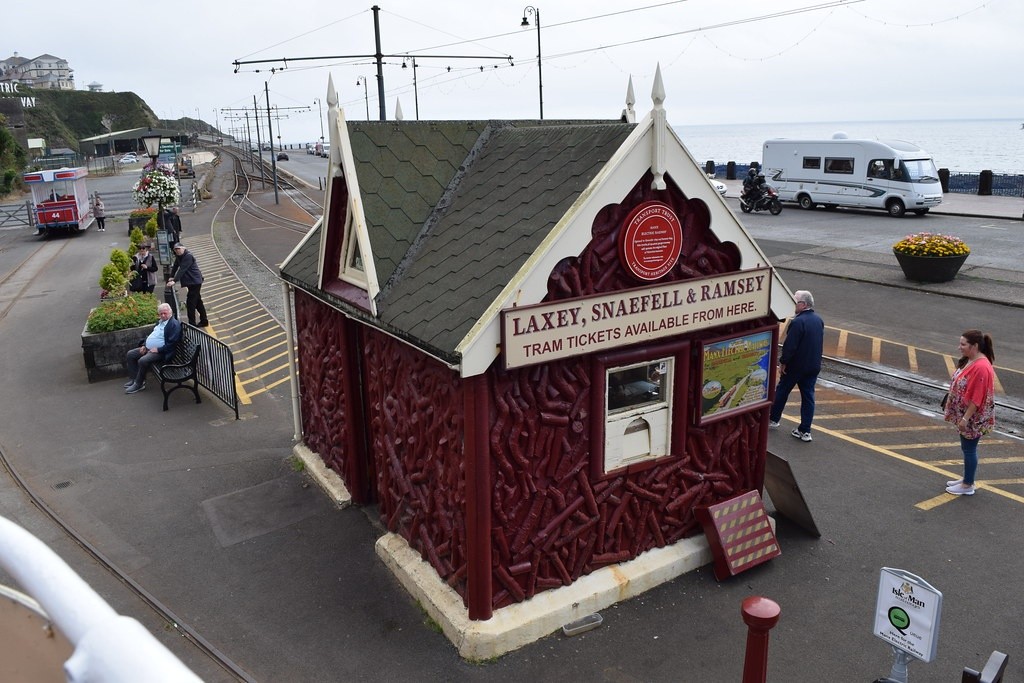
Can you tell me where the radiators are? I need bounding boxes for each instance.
[129,207,154,217]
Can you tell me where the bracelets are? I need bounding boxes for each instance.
[963,418,968,422]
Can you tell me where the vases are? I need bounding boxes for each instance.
[81,300,162,384]
[893,247,970,283]
[127,217,151,236]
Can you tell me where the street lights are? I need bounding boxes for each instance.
[313,97,325,143]
[139,124,179,293]
[213,107,219,130]
[520,4,545,121]
[356,74,370,120]
[194,105,202,136]
[402,53,421,121]
[271,103,283,151]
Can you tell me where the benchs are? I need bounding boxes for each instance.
[138,334,202,411]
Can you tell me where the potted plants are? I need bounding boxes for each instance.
[129,225,144,250]
[144,217,158,246]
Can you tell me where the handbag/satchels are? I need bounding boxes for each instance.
[940,392,950,411]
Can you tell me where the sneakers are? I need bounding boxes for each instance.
[945,484,975,495]
[945,478,976,488]
[791,428,812,441]
[126,384,145,393]
[769,420,780,428]
[124,380,146,387]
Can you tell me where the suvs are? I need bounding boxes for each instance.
[192,132,198,137]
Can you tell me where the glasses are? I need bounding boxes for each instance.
[139,247,146,250]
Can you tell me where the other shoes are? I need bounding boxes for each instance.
[102,229,105,232]
[97,229,101,232]
[196,319,209,327]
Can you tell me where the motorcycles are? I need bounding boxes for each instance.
[739,182,783,215]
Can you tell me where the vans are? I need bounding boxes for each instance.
[761,130,944,218]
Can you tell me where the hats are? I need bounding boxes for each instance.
[136,241,151,248]
[173,242,184,250]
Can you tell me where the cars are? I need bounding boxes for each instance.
[276,152,289,161]
[216,137,223,142]
[307,142,330,159]
[234,137,241,143]
[709,178,728,200]
[119,151,150,165]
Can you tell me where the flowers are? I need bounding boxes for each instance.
[86,291,161,333]
[131,161,181,210]
[893,232,970,257]
[98,248,139,303]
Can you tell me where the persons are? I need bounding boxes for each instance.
[124,302,183,394]
[742,168,766,203]
[50,189,60,201]
[768,290,824,441]
[167,242,208,327]
[93,197,106,231]
[130,242,158,295]
[157,206,183,245]
[943,329,995,496]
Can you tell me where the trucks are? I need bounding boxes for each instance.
[249,143,259,152]
[262,142,271,151]
